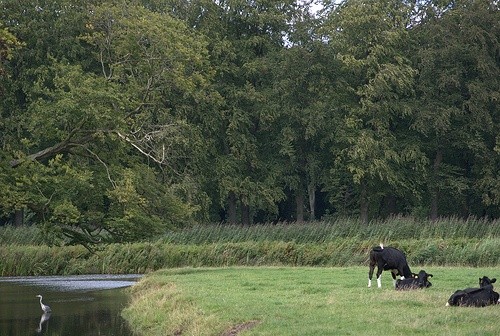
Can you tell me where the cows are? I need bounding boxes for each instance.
[367,242,415,288]
[445,276,500,307]
[395,270,433,291]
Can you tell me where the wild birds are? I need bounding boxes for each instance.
[35,295,52,312]
[35,313,51,333]
